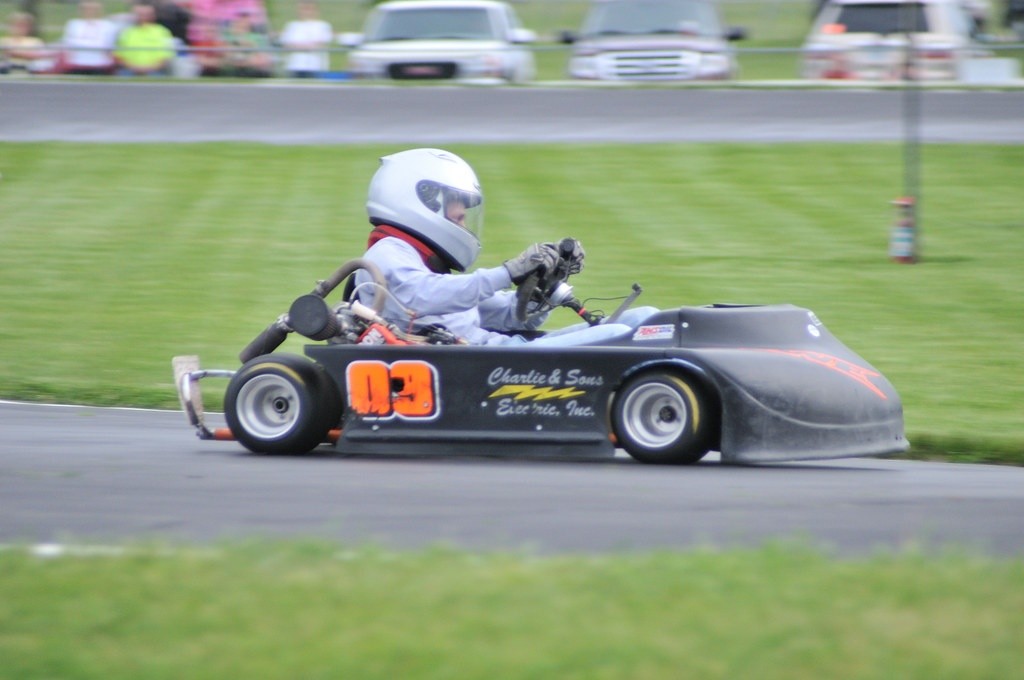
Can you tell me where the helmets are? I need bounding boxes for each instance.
[364,146,482,273]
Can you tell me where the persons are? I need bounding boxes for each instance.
[356,148,657,347]
[112,3,177,76]
[0,11,44,72]
[192,11,276,77]
[280,0,332,78]
[62,3,118,74]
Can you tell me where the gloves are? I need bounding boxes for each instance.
[564,238,583,274]
[503,241,560,280]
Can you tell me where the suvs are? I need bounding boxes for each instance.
[557,1,751,83]
[340,0,537,86]
[802,0,976,84]
